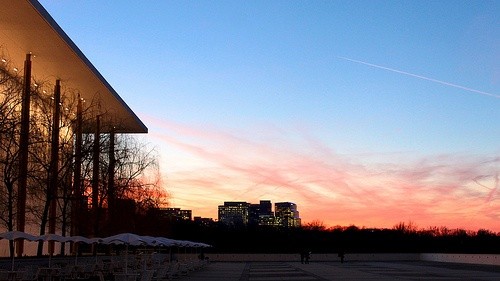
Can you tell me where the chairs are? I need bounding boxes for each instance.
[0,255,211,281]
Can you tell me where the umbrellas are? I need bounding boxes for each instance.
[35,233,68,267]
[90,233,212,273]
[66,236,94,266]
[0,230,35,272]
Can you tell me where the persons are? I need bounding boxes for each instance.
[337,246,345,263]
[298,245,312,264]
